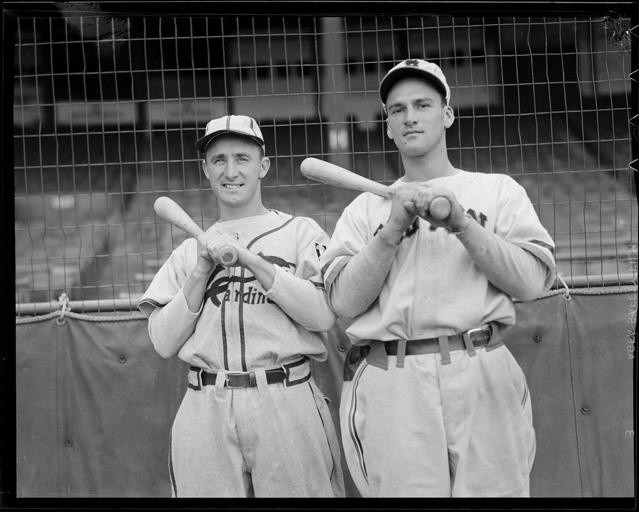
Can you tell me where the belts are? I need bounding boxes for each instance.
[385,326,490,354]
[201,368,284,389]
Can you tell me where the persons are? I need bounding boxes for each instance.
[320,57,558,499]
[138,113,345,499]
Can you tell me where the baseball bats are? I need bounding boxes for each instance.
[300,157,451,220]
[154,196,238,267]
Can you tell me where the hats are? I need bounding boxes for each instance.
[194,114,267,158]
[377,57,452,116]
